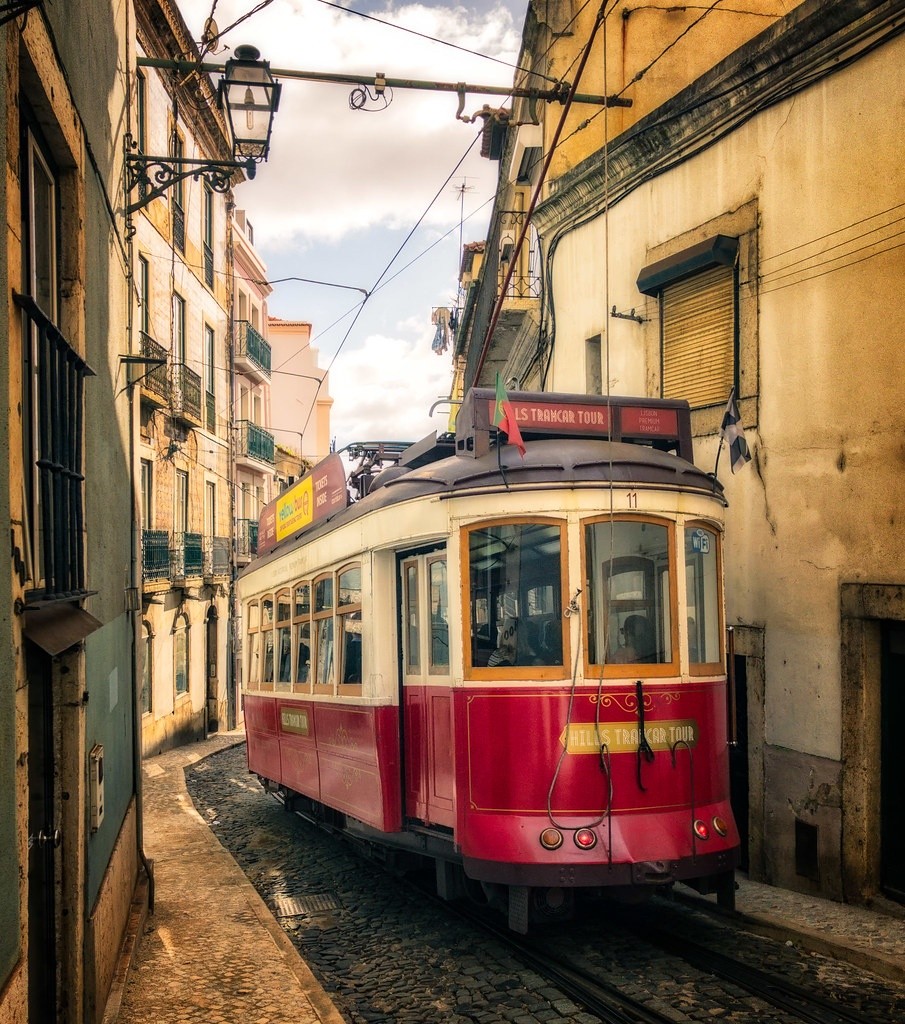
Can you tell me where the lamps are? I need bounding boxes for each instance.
[123,43,282,242]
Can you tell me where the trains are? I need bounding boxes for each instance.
[235,387,741,941]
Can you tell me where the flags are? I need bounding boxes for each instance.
[492,372,526,460]
[717,385,752,476]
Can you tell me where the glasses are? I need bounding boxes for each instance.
[619,628,628,634]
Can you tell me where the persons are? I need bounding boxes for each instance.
[605,614,656,664]
[471,620,562,667]
[265,612,360,684]
